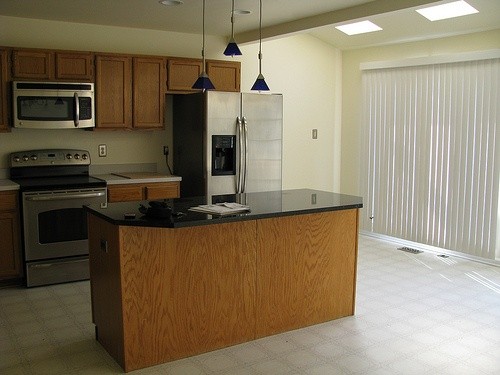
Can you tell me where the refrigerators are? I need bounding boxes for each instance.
[173,90,284,196]
[172,191,283,221]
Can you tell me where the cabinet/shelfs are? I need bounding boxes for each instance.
[0,46,95,134]
[107,181,181,204]
[166,56,241,94]
[87,52,166,131]
[0,189,24,286]
[82,188,365,373]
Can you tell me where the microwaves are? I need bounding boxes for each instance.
[8,78,96,130]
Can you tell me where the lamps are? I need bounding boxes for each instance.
[251,0,270,90]
[223,0,242,55]
[192,0,217,89]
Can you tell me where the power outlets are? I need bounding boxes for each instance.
[163,145,169,156]
[98,144,107,157]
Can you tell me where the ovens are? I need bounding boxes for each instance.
[19,187,107,288]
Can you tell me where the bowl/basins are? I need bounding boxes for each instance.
[138,206,172,215]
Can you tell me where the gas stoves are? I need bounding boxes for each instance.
[8,148,108,192]
